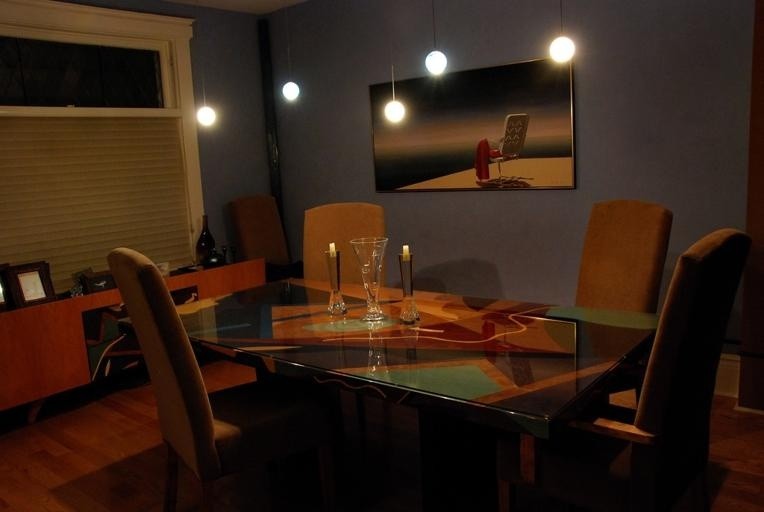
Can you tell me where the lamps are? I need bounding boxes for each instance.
[425,0,447,76]
[282,6,300,101]
[384,0,405,123]
[195,6,217,127]
[548,0,575,63]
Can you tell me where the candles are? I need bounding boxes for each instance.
[402,245,410,262]
[328,241,337,257]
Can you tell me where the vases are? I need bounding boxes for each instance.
[349,237,386,319]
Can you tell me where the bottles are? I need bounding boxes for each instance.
[195,214,216,260]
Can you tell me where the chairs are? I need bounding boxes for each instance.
[302,202,385,465]
[575,200,674,411]
[107,246,341,511]
[532,226,752,512]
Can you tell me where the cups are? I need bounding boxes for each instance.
[397,252,422,325]
[348,236,390,322]
[324,249,348,316]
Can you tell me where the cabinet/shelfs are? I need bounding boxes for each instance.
[0,256,266,411]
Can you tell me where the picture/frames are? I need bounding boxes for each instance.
[0,263,18,312]
[368,57,577,193]
[9,260,57,308]
